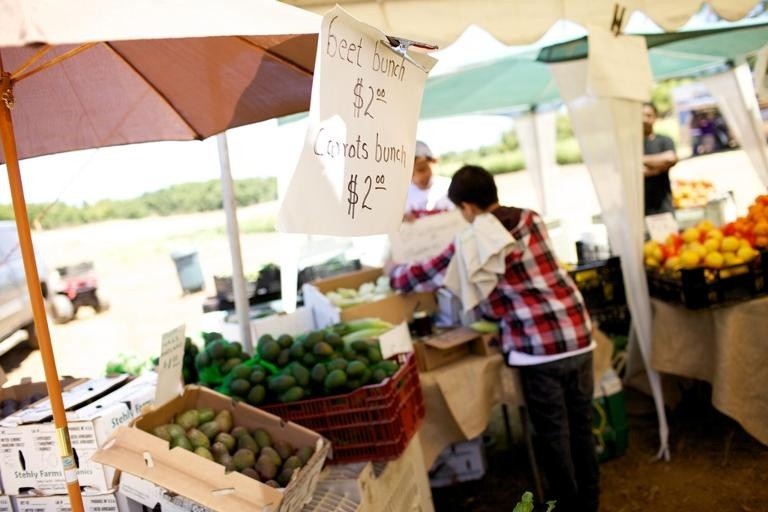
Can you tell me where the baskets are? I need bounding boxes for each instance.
[644,247,767,310]
[255,338,429,465]
[566,256,631,338]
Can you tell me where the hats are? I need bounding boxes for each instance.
[414,141,438,163]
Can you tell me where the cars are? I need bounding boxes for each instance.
[0,220,41,364]
[41,248,112,323]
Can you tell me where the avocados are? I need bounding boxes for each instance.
[182,329,399,405]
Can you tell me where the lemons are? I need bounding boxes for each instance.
[645,220,756,284]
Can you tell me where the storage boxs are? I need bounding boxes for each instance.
[223,268,501,372]
[0,373,332,512]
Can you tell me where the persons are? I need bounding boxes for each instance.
[384,164,599,512]
[644,102,678,216]
[692,110,730,153]
[383,140,461,275]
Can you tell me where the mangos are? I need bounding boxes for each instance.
[149,408,312,488]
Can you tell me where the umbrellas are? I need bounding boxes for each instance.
[1,1,440,511]
